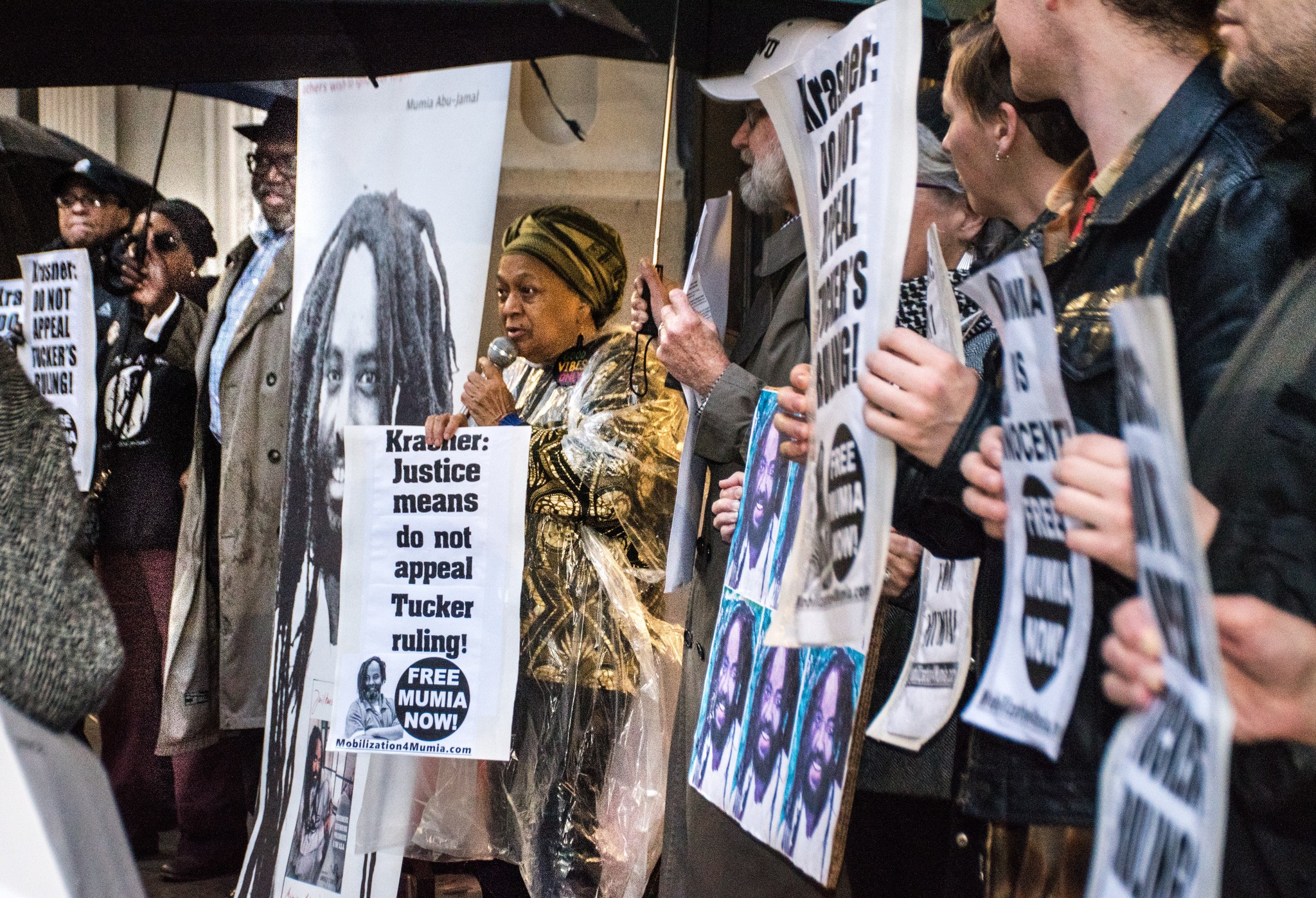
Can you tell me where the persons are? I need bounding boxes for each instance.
[775,651,852,886]
[726,404,791,605]
[689,602,756,810]
[154,94,297,757]
[345,656,404,741]
[425,204,712,898]
[238,192,458,898]
[37,159,249,882]
[285,726,333,884]
[773,0,1316,898]
[630,20,849,898]
[728,646,801,843]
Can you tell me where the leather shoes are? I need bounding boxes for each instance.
[158,828,248,879]
[128,834,159,859]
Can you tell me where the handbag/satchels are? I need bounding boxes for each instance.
[75,470,115,568]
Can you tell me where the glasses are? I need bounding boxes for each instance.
[247,151,297,179]
[744,105,770,129]
[56,193,119,209]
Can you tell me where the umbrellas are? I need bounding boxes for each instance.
[0,0,660,291]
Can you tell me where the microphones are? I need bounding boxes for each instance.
[459,337,515,419]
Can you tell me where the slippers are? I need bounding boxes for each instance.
[150,231,191,254]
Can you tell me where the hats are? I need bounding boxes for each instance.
[232,94,298,143]
[697,18,850,104]
[53,159,136,207]
[500,205,625,330]
[137,198,218,270]
